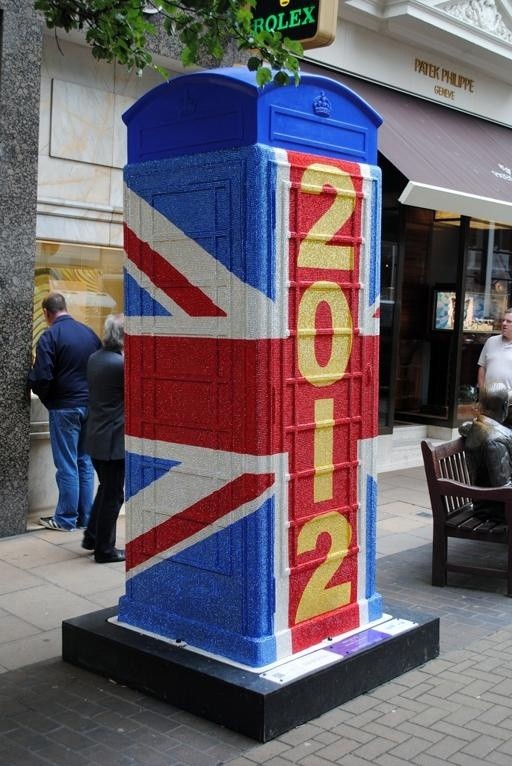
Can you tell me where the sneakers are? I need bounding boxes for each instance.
[39,516,87,532]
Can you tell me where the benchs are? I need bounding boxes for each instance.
[421,436,512,595]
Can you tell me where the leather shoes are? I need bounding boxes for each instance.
[81,536,126,563]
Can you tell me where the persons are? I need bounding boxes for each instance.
[477,308,512,406]
[81,313,125,563]
[464,380,512,522]
[28,293,103,532]
